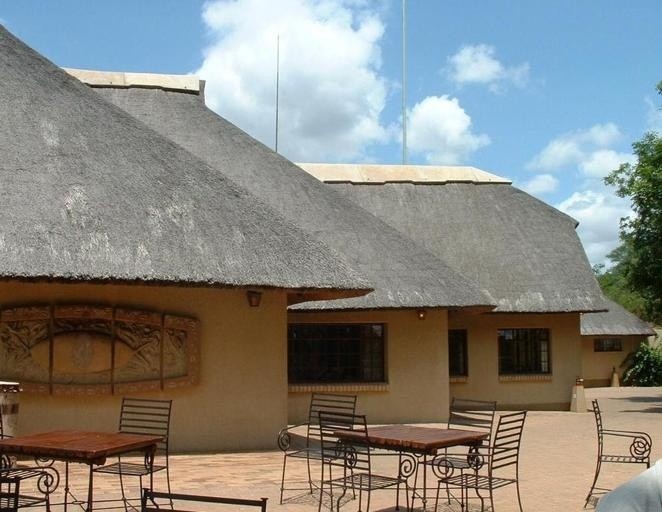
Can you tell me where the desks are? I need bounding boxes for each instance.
[0,429,165,512]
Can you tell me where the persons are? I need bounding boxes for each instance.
[595,459,661,512]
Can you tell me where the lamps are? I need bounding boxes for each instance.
[246,290,262,306]
[417,310,426,320]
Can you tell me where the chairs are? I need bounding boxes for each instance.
[0,408,50,512]
[584,398,652,507]
[277,390,528,512]
[140,488,268,512]
[87,397,174,512]
